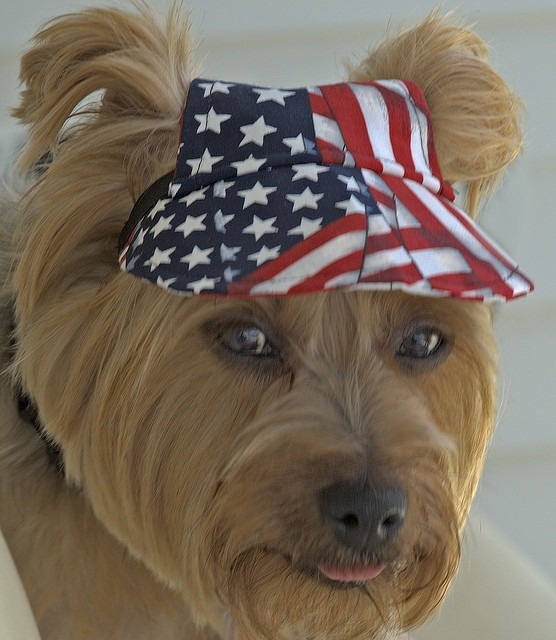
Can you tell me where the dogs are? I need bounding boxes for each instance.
[0,0,537,640]
